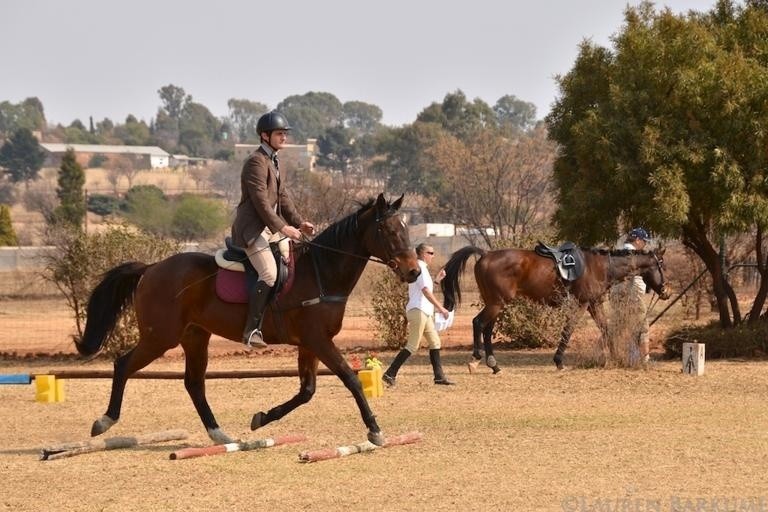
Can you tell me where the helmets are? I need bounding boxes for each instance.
[255,111,294,135]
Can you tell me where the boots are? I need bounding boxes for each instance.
[242,281,276,350]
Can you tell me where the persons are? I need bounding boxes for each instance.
[232,111,315,349]
[610,228,650,373]
[382,243,458,386]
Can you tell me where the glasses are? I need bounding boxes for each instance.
[424,252,434,255]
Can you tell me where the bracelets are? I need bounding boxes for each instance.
[434,280,440,285]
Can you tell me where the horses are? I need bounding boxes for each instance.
[66,189,423,450]
[440,246,674,380]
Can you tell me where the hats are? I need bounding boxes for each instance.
[629,227,652,242]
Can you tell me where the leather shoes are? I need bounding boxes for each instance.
[381,374,396,386]
[433,377,456,385]
